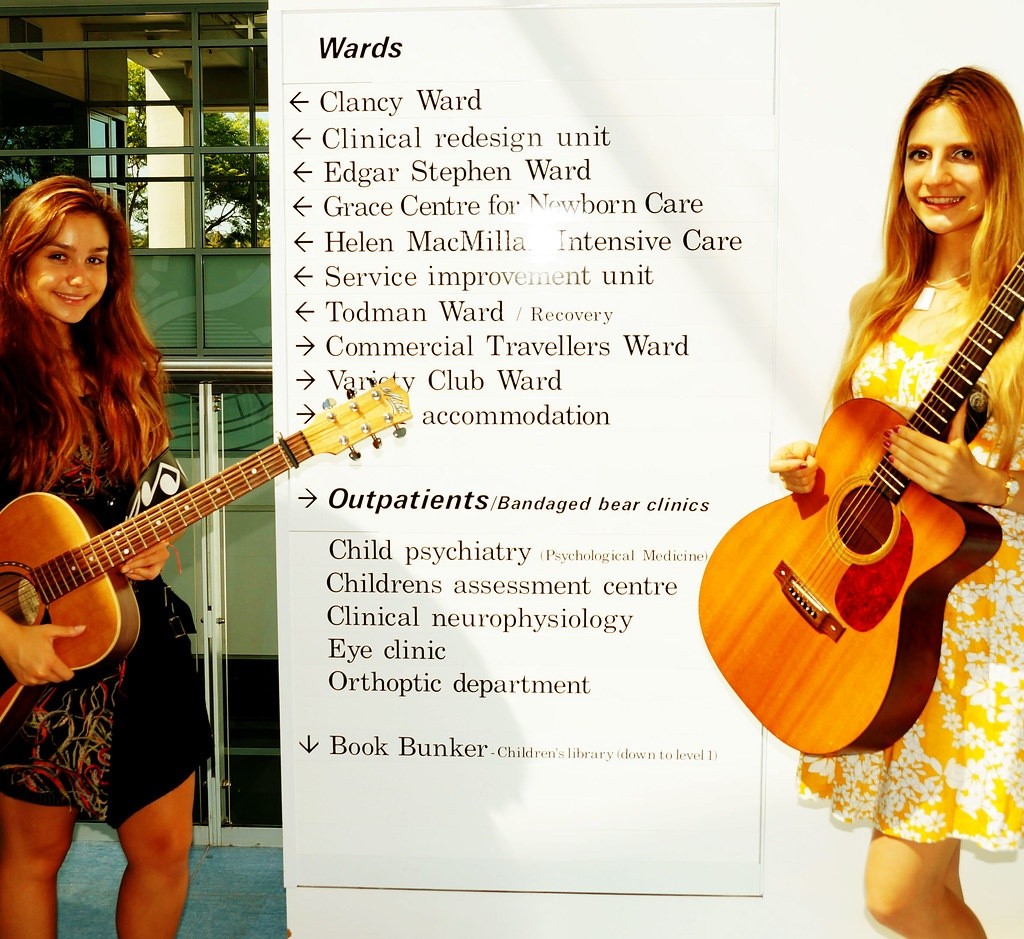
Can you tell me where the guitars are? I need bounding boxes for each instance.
[696,249,1024,757]
[0,376,414,721]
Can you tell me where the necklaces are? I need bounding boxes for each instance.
[912,269,971,311]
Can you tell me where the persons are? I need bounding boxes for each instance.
[0,174,216,939]
[769,67,1024,939]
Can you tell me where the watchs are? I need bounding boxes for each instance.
[992,469,1020,508]
